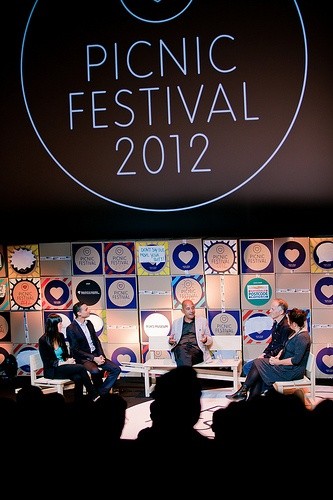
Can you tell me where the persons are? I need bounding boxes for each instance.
[66,302,121,395]
[240,298,295,394]
[226,308,310,401]
[0,364,332,500]
[168,299,213,368]
[39,313,100,395]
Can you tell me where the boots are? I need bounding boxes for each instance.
[226,385,248,401]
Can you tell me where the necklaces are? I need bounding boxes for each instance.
[282,325,304,353]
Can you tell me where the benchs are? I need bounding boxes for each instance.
[274,352,316,407]
[143,335,242,397]
[30,343,101,395]
